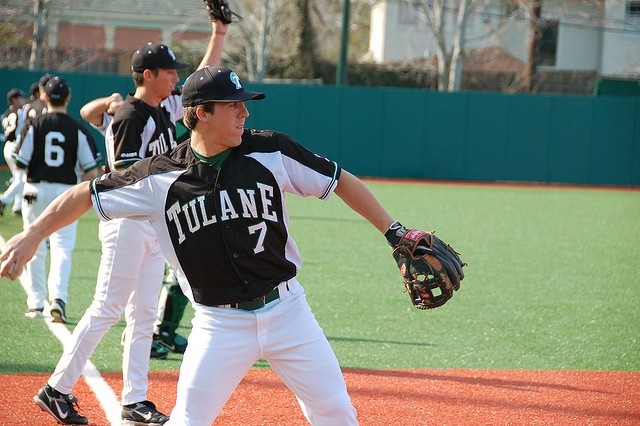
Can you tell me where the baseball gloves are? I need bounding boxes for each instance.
[391,227,464,310]
[201,0,232,24]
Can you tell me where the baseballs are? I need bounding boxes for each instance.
[0,259,23,280]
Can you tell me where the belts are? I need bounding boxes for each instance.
[216,281,289,311]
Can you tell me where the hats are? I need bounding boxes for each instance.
[181,65,266,106]
[30,82,39,96]
[38,73,51,87]
[45,76,68,98]
[131,42,189,72]
[7,87,28,100]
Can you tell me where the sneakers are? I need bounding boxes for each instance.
[50,300,66,323]
[33,382,88,425]
[121,400,168,426]
[24,308,42,318]
[150,341,169,359]
[155,330,188,354]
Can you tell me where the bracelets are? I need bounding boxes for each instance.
[212,30,227,35]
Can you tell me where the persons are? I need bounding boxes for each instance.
[12,76,105,323]
[1,66,463,426]
[29,81,40,101]
[21,73,51,121]
[33,42,189,426]
[79,0,231,359]
[0,88,25,214]
